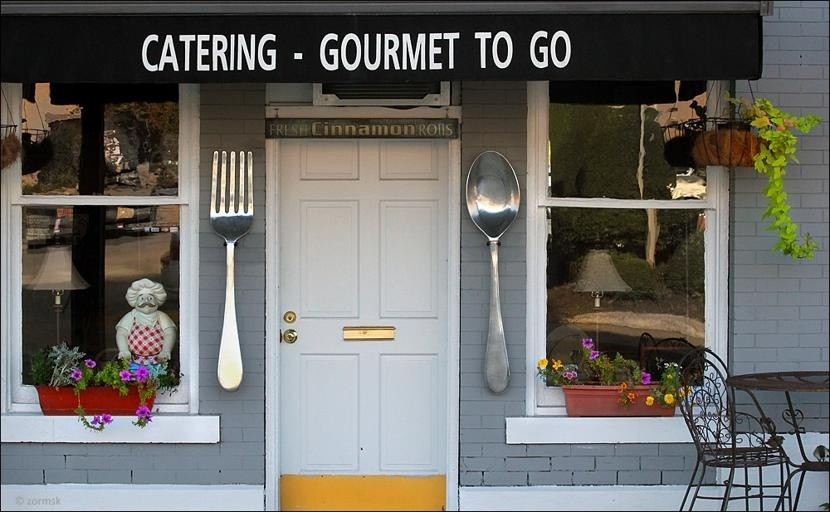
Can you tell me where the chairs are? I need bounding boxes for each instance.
[674,345,794,511]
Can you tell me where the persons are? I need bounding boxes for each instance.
[113,275,175,371]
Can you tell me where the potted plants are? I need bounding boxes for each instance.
[660,90,824,263]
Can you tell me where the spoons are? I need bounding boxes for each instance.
[465,148,525,397]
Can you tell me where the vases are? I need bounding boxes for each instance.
[35,385,158,416]
[558,382,681,416]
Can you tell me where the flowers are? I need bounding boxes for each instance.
[535,334,693,412]
[35,342,182,432]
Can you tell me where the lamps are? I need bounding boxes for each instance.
[566,247,634,351]
[24,244,90,340]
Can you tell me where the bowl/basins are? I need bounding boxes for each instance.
[547,324,595,383]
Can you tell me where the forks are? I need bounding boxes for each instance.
[206,146,257,395]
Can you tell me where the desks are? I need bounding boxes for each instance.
[639,342,688,376]
[723,368,829,511]
